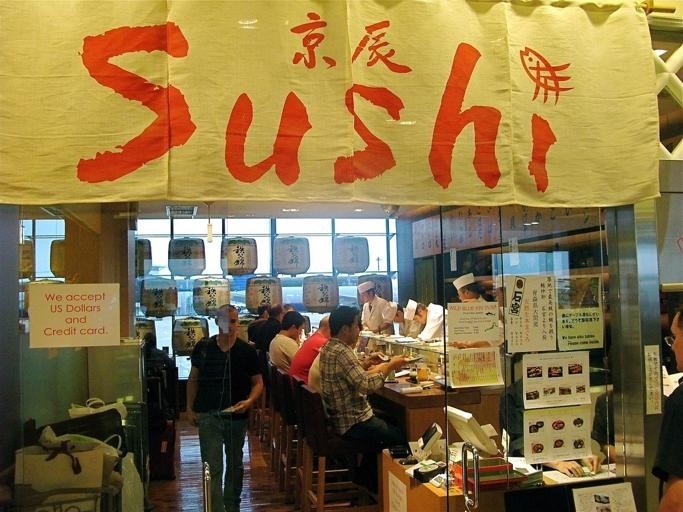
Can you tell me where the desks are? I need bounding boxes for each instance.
[353,349,482,453]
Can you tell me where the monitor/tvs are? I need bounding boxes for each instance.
[441,403,500,461]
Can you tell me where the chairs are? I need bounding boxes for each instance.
[242,350,376,510]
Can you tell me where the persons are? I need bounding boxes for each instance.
[382,300,424,338]
[184,303,265,511]
[143,333,174,376]
[249,303,410,504]
[358,280,394,334]
[651,309,683,511]
[452,271,616,477]
[404,298,448,342]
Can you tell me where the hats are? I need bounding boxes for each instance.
[453,273,474,290]
[402,299,418,321]
[357,281,375,294]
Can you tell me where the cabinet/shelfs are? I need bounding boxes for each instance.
[445,229,610,321]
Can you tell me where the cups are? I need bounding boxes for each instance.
[416,362,432,381]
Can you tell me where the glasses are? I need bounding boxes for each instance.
[663,333,677,347]
